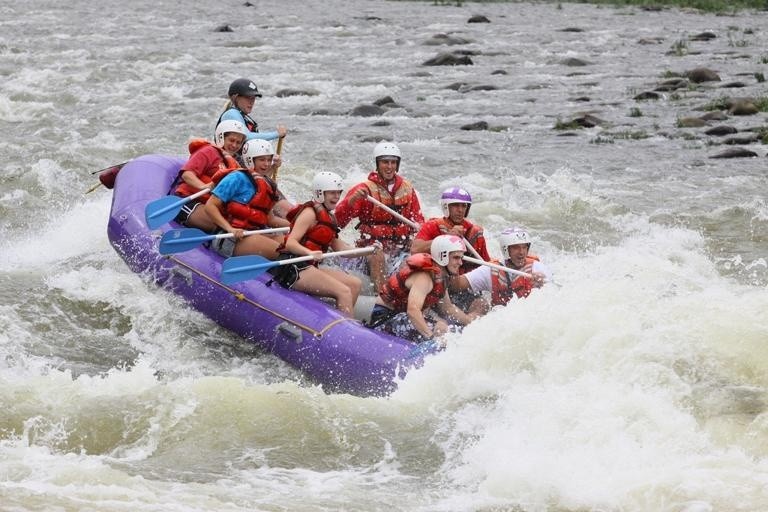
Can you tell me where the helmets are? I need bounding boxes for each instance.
[215,120,246,150]
[243,139,274,172]
[429,235,466,268]
[440,187,473,219]
[499,227,532,261]
[312,171,346,204]
[227,78,262,99]
[371,141,402,174]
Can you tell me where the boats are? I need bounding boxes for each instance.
[100,153,490,404]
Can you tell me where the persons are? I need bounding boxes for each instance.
[335,141,425,294]
[214,79,286,155]
[203,139,290,260]
[410,187,491,317]
[173,120,282,234]
[272,171,383,318]
[370,234,473,343]
[445,232,552,309]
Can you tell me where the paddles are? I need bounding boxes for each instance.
[221,245,376,284]
[158,227,292,255]
[145,187,211,229]
